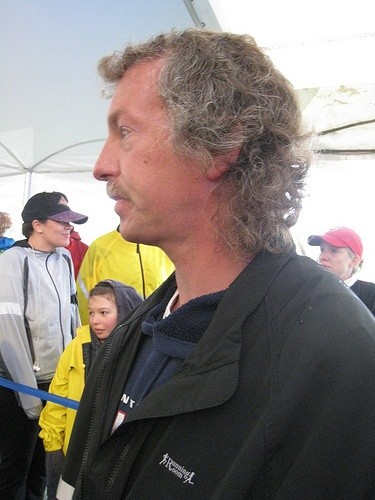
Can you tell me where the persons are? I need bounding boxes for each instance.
[75,225,175,325]
[52,28,375,500]
[38,280,145,500]
[307,226,375,316]
[0,192,88,500]
[0,212,16,251]
[66,229,88,278]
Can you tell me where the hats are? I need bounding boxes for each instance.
[308,226,364,257]
[21,191,88,226]
